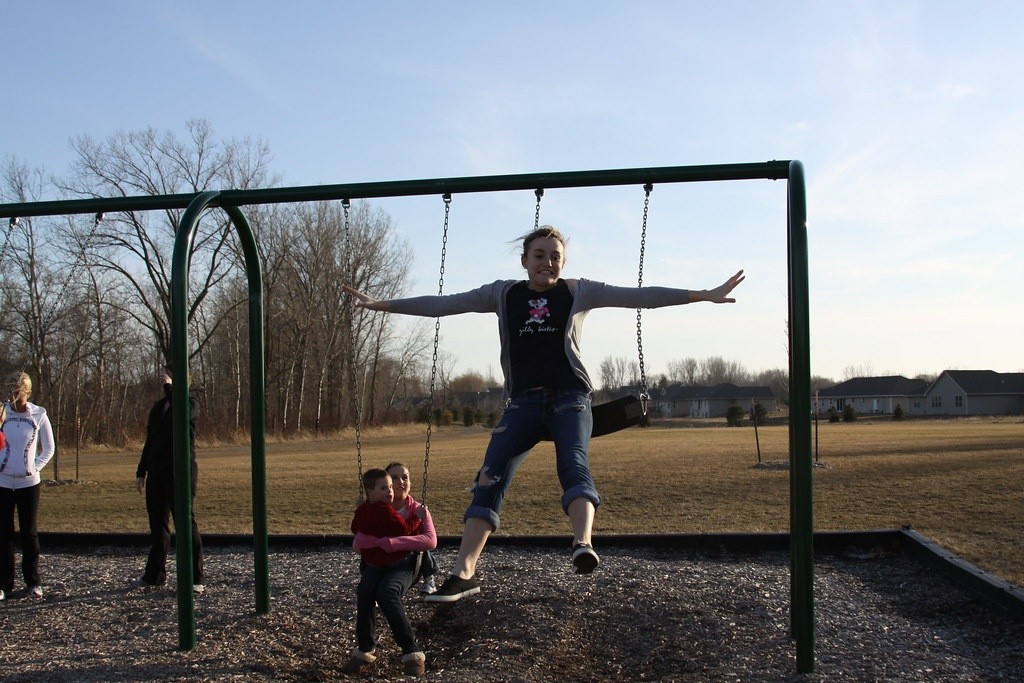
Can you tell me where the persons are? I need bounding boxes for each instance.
[135,361,206,592]
[0,372,55,601]
[343,227,745,602]
[342,462,436,678]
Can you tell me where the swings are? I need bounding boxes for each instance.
[526,190,654,440]
[0,222,98,410]
[343,203,454,587]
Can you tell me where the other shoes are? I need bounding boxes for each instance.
[0,589,13,600]
[342,647,377,674]
[131,580,166,588]
[26,584,43,597]
[426,573,481,602]
[403,652,426,677]
[424,580,436,593]
[194,584,206,592]
[571,542,600,576]
